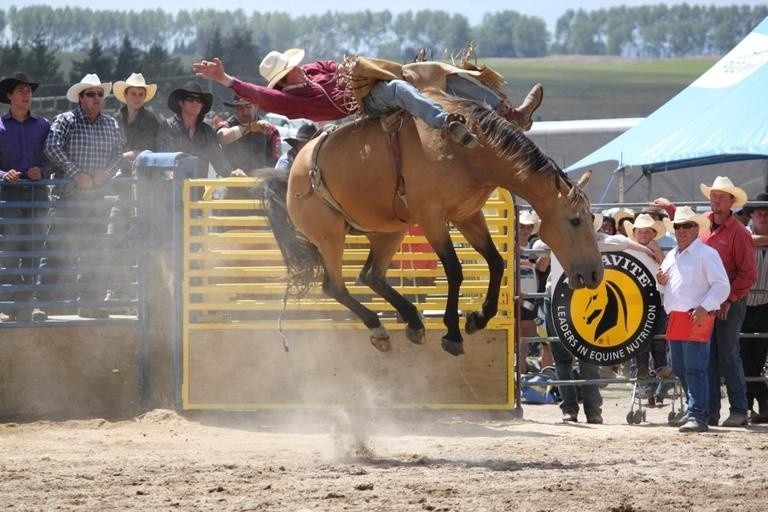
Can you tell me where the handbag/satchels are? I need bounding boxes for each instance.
[518,366,583,405]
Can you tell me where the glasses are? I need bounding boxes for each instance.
[673,223,698,230]
[181,94,204,104]
[82,89,105,98]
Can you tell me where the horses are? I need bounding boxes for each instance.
[258,83,606,359]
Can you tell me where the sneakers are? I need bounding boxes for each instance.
[29,306,50,321]
[77,305,112,320]
[586,414,604,424]
[1,308,16,323]
[638,364,673,379]
[668,411,720,432]
[722,409,768,427]
[559,411,579,424]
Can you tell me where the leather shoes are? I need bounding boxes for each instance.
[446,119,478,150]
[511,84,543,133]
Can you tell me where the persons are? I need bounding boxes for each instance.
[385,226,437,315]
[517,175,767,434]
[0,69,318,322]
[191,49,547,134]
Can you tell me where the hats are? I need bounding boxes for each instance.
[699,176,747,208]
[167,80,214,115]
[0,73,39,104]
[518,209,540,235]
[66,73,112,103]
[283,122,317,150]
[259,47,305,88]
[223,93,250,107]
[113,73,157,104]
[663,208,711,234]
[591,207,669,240]
[743,193,768,220]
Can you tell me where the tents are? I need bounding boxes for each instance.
[560,12,768,203]
